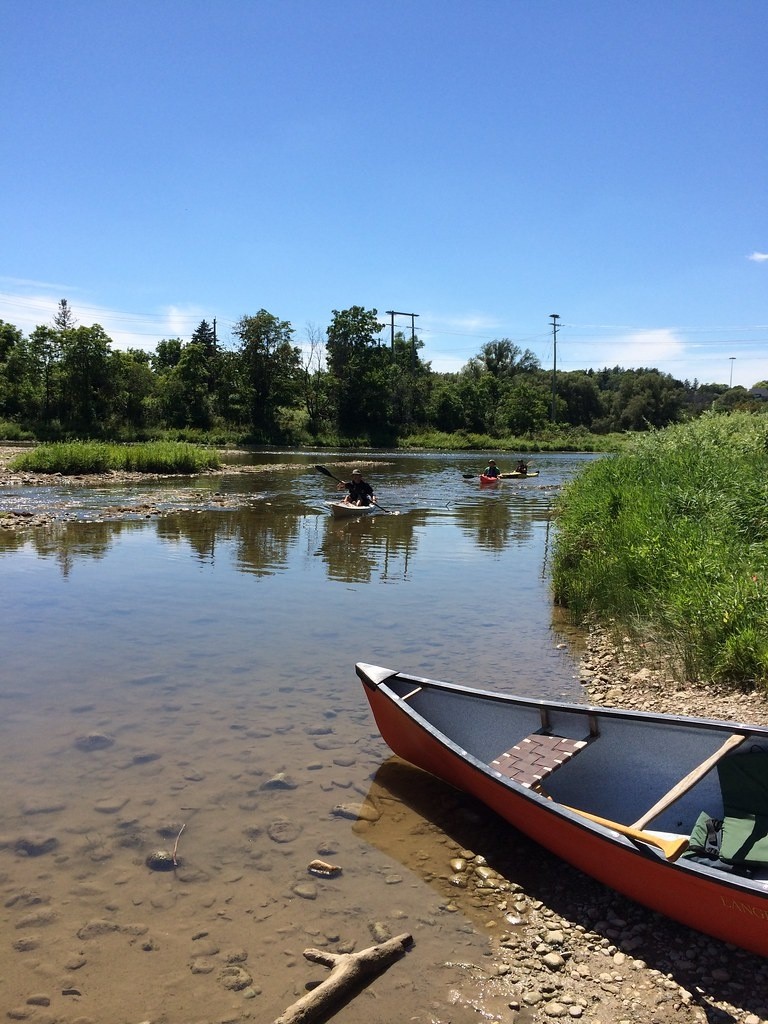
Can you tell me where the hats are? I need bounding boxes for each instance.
[518,459,523,463]
[350,469,362,475]
[488,459,496,465]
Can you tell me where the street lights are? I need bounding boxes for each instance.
[729,357,737,389]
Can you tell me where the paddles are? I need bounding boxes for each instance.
[313,464,393,512]
[462,474,519,479]
[557,803,690,860]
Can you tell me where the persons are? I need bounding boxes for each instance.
[337,469,376,506]
[516,459,528,474]
[483,459,500,478]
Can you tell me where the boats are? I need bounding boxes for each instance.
[322,496,377,517]
[479,474,500,485]
[498,470,540,479]
[354,661,767,959]
